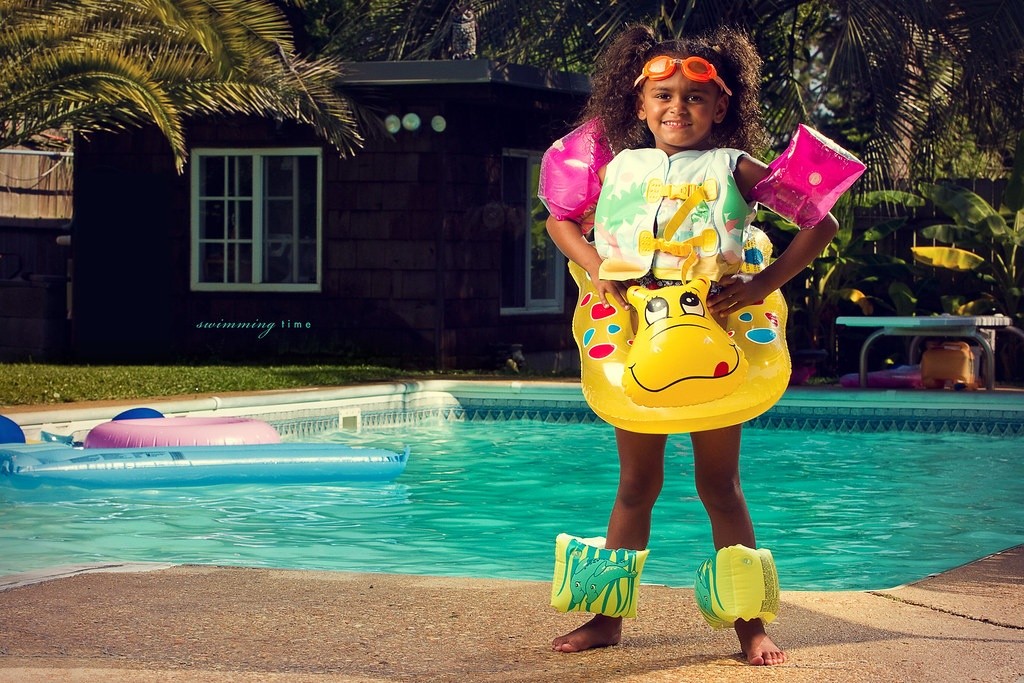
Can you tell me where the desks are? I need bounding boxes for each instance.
[835,316,1013,390]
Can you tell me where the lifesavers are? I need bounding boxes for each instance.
[537,117,615,234]
[749,124,866,231]
[694,544,781,628]
[85,416,282,447]
[567,225,793,435]
[552,532,649,619]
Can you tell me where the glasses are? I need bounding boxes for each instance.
[642,56,716,83]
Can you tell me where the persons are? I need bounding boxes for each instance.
[547,25,839,664]
